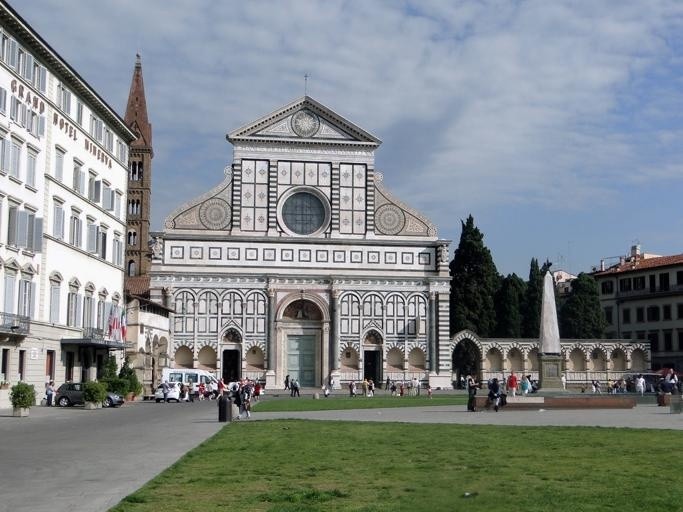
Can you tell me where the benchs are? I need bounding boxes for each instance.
[474,394,502,410]
[543,394,637,408]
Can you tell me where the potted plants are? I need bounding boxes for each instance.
[81,379,108,410]
[122,375,143,401]
[8,380,37,418]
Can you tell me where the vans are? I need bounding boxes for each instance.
[161,368,226,399]
[613,373,661,391]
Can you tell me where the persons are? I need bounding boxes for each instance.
[591,368,678,396]
[162,377,261,420]
[466,370,538,411]
[349,376,432,397]
[321,377,334,398]
[41,381,55,407]
[283,375,301,398]
[561,373,565,389]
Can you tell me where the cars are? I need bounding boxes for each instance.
[56,383,124,407]
[155,382,190,403]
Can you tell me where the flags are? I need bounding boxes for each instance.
[108,304,127,339]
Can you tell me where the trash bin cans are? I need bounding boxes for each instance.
[219,390,232,422]
